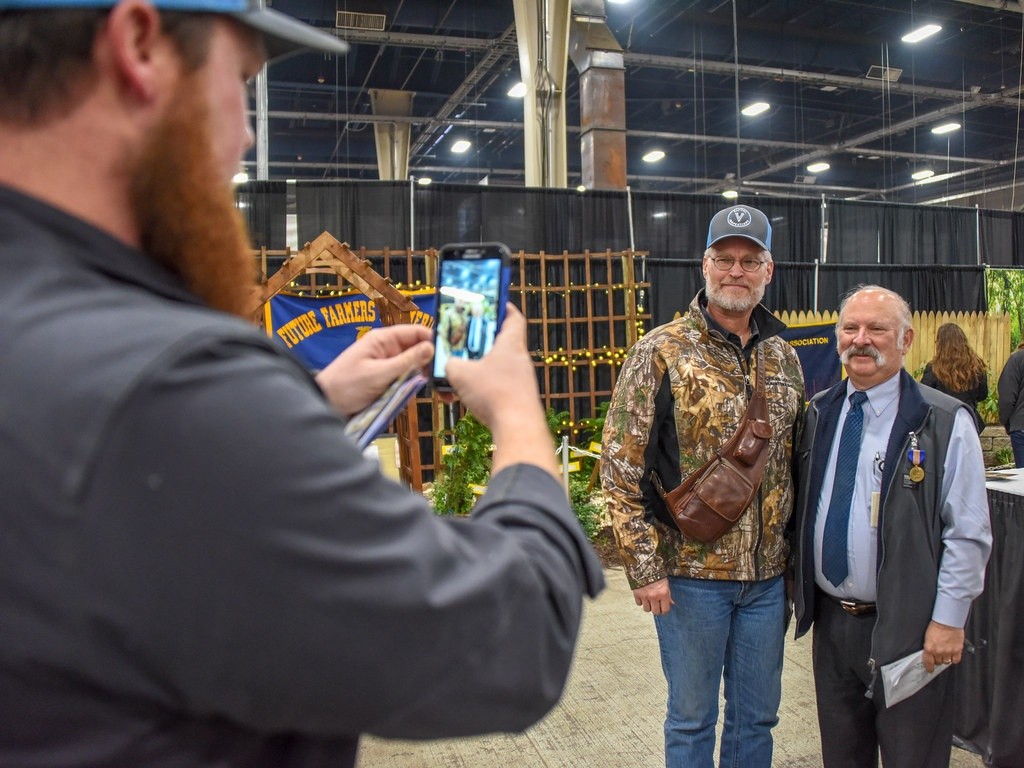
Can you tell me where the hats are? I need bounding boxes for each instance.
[0,0,349,59]
[706,204,772,252]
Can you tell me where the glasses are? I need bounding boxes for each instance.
[708,255,765,271]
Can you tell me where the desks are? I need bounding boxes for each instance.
[952,467,1024,768]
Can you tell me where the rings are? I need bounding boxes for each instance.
[942,659,951,664]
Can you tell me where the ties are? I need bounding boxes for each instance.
[822,392,868,588]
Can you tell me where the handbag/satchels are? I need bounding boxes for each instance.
[648,340,772,544]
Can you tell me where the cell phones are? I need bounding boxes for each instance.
[431,240,511,392]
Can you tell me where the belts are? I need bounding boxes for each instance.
[817,592,876,617]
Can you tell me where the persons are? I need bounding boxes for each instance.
[997,330,1024,469]
[0,0,607,768]
[601,204,807,768]
[440,296,488,360]
[793,282,994,767]
[920,323,989,437]
